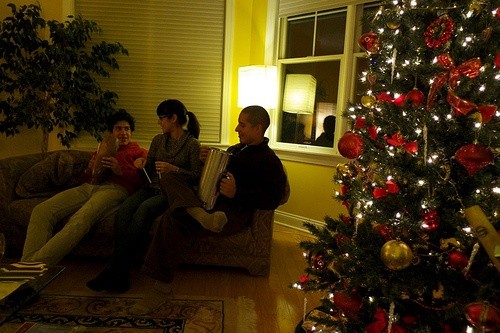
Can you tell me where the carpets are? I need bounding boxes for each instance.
[0,290,238,333]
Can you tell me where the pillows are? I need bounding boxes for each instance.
[16,153,74,198]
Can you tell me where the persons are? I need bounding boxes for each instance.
[129,106,287,315]
[21,108,148,267]
[313,116,336,148]
[86,99,202,294]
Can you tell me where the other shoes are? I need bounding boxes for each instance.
[86,265,130,293]
[130,282,174,315]
[196,211,227,233]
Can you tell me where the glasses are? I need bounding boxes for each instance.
[159,115,167,121]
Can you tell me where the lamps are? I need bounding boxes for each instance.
[282,73,318,142]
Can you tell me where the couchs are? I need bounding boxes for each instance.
[0,149,290,276]
